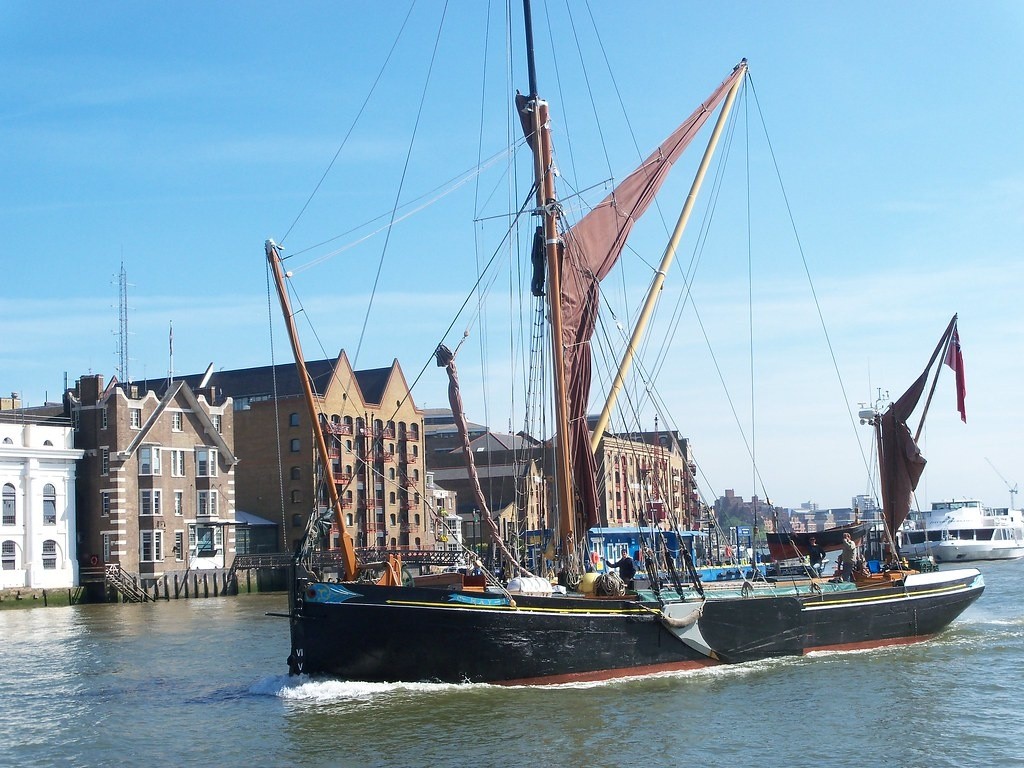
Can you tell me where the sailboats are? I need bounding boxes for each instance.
[238,0,988,688]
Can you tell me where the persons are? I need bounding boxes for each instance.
[469,560,482,576]
[807,536,826,578]
[838,533,857,583]
[600,549,636,589]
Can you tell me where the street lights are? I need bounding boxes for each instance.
[476,509,483,563]
[704,506,713,559]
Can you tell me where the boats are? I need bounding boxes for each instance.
[897,496,1024,560]
[518,525,767,590]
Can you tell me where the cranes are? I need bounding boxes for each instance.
[984,456,1019,510]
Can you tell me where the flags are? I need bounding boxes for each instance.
[943,318,967,424]
